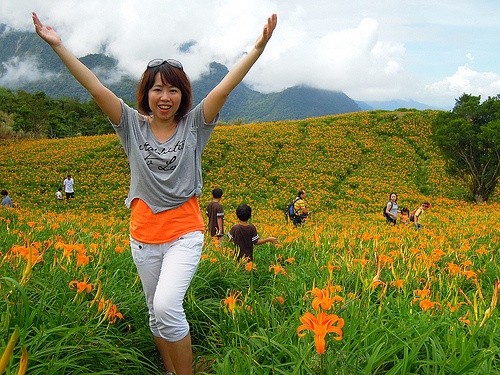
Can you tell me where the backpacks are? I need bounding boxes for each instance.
[383,202,393,217]
[290,203,294,216]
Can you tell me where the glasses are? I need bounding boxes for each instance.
[148,58,183,70]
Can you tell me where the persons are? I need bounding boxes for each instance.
[0,190,12,207]
[386,192,401,225]
[55,188,63,200]
[226,204,277,263]
[63,175,74,198]
[400,207,410,223]
[206,188,224,239]
[410,202,431,226]
[293,190,309,226]
[33,13,277,375]
